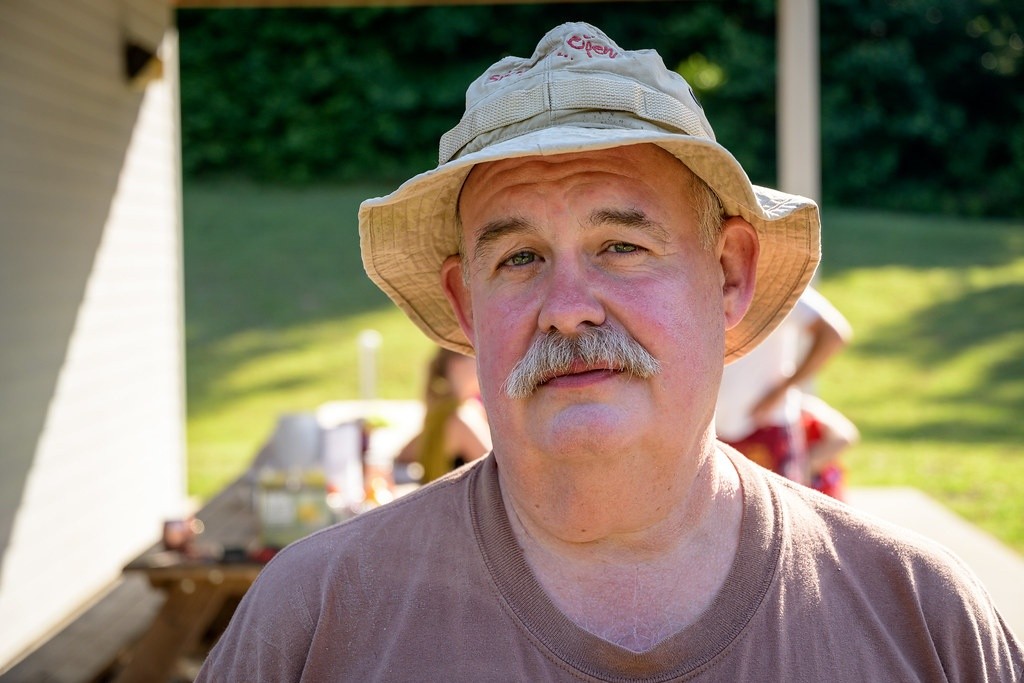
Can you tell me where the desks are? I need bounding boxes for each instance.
[121,399,488,681]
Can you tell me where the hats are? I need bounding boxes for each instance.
[358,21,822,368]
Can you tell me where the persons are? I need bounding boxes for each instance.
[397,346,490,481]
[717,285,857,502]
[195,20,1024,683]
